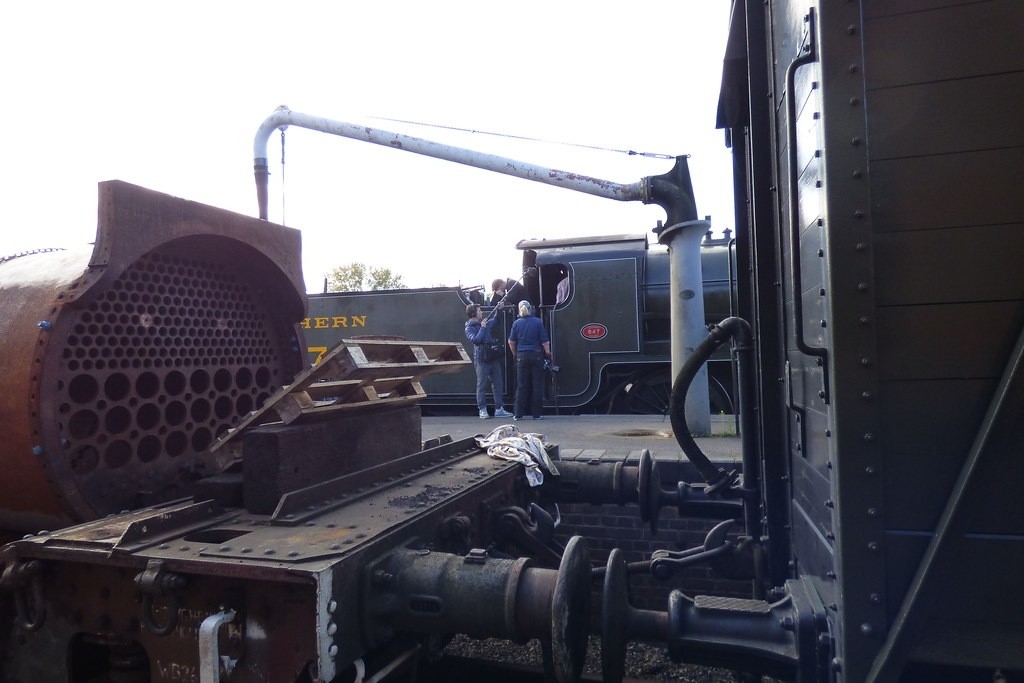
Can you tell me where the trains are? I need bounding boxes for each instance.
[287,233,738,417]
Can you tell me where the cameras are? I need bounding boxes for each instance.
[543,359,559,373]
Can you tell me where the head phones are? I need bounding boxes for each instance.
[470,304,477,317]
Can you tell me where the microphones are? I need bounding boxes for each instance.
[528,268,538,276]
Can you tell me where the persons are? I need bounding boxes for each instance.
[508,300,550,420]
[489,278,530,305]
[464,303,513,419]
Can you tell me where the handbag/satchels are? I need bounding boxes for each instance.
[481,342,505,362]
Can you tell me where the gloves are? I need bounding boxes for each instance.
[545,352,551,364]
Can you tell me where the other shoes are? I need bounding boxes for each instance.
[513,416,524,421]
[494,407,513,419]
[533,415,542,420]
[479,406,489,418]
